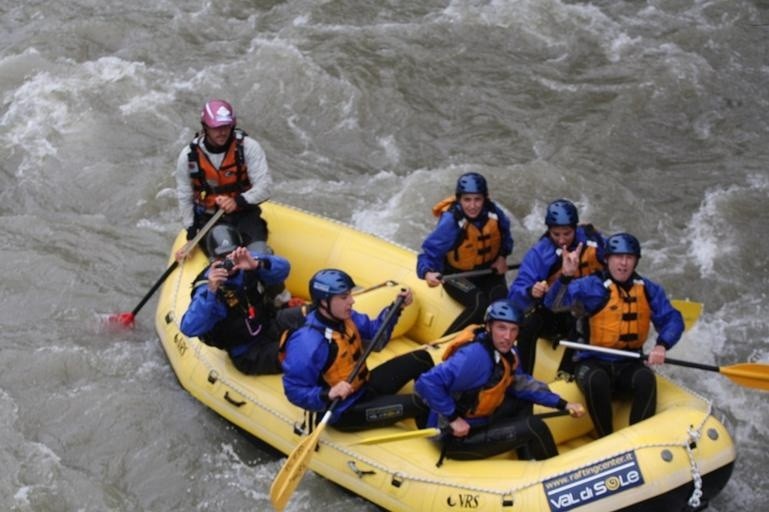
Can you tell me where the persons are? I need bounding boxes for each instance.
[283,270,436,432]
[544,233,685,438]
[416,171,514,340]
[180,226,312,374]
[173,99,275,258]
[414,301,585,459]
[508,200,605,377]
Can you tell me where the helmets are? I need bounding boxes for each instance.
[456,172,488,202]
[309,269,355,308]
[207,224,243,258]
[484,301,525,328]
[605,234,641,258]
[200,100,237,129]
[546,199,579,226]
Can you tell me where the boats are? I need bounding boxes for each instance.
[153,199,736,512]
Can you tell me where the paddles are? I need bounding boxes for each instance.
[268,286,410,511]
[554,338,769,393]
[114,207,223,331]
[345,406,585,447]
[669,296,705,334]
